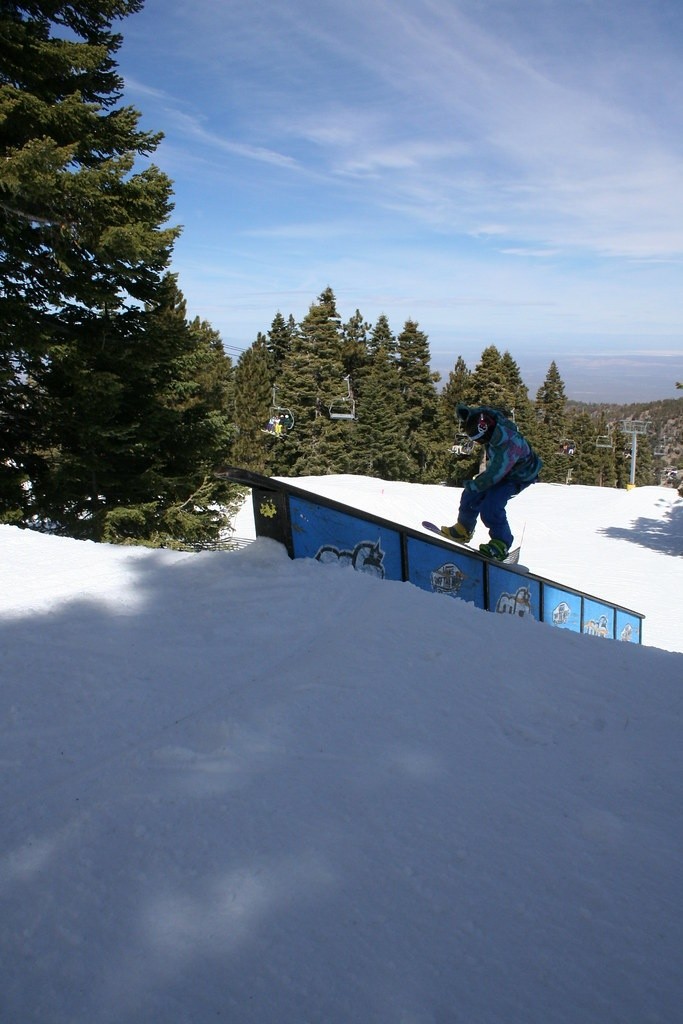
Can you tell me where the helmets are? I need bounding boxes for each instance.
[464,412,495,444]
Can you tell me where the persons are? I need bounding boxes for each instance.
[266,414,291,436]
[442,405,543,562]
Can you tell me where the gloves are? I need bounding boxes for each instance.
[463,481,479,494]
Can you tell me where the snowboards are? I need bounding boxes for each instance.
[422,520,530,574]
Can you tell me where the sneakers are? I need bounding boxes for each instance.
[441,523,475,543]
[480,538,509,561]
